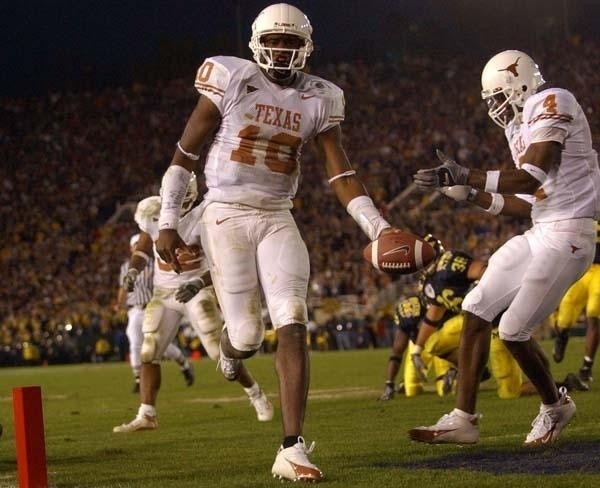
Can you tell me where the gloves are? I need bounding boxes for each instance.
[175,277,206,304]
[409,353,433,384]
[378,380,397,403]
[412,147,473,195]
[121,267,141,294]
[435,365,458,397]
[435,175,475,205]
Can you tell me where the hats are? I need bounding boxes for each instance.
[128,233,140,249]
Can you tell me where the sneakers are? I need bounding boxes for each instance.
[481,366,493,385]
[215,335,240,382]
[552,335,569,364]
[181,361,196,389]
[579,365,595,385]
[519,385,579,451]
[112,412,160,434]
[248,388,276,424]
[132,378,142,395]
[407,408,482,447]
[564,371,592,392]
[269,435,327,483]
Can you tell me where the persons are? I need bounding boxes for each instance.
[1,33,600,367]
[113,171,274,433]
[380,50,598,447]
[157,4,402,483]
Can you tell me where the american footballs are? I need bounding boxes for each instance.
[363,231,435,275]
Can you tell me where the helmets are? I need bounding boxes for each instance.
[421,231,446,262]
[247,2,316,53]
[477,47,550,110]
[159,169,200,202]
